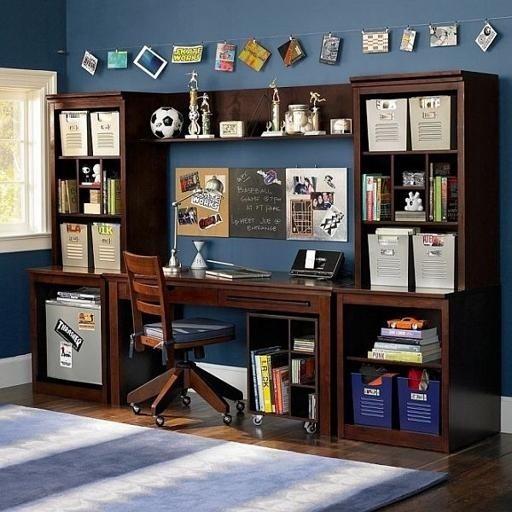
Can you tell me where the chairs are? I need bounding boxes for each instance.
[117,247,246,428]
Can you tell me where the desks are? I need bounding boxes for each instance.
[102,269,335,435]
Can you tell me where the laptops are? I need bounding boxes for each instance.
[206,259,272,279]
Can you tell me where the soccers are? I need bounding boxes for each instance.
[150,107,183,139]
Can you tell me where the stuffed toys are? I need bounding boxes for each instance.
[81,166,92,181]
[91,163,101,182]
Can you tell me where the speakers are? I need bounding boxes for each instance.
[288,248,345,278]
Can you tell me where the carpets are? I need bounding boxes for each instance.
[1,401,450,510]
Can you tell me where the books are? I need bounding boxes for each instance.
[250,335,316,420]
[57,178,122,215]
[57,286,101,306]
[363,161,459,224]
[366,326,441,363]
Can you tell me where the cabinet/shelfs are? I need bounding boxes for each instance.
[336,78,503,445]
[146,79,353,147]
[24,86,158,406]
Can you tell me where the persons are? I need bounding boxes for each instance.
[219,51,236,62]
[291,175,335,211]
